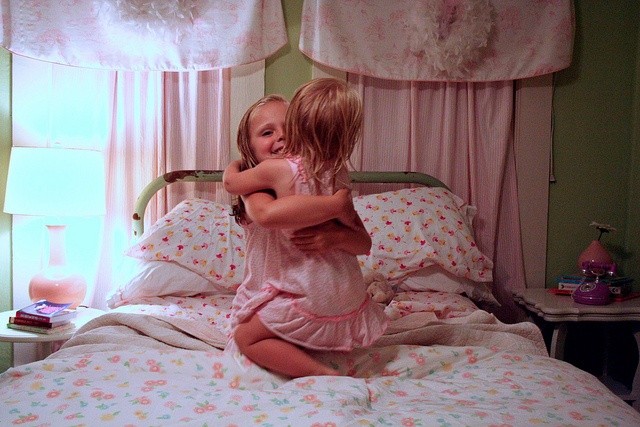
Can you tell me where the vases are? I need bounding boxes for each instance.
[577,241,615,274]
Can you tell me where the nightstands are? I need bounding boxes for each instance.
[512,287,639,406]
[0,310,103,365]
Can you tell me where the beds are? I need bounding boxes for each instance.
[0,168,640,427]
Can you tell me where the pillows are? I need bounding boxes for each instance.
[106,260,499,309]
[124,188,493,286]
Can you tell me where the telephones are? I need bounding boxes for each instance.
[570,261,618,307]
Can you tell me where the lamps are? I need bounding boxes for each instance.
[3,143,106,308]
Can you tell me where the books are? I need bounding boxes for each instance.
[552,274,640,297]
[6,301,79,335]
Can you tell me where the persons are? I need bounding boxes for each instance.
[223,78,373,377]
[228,95,371,338]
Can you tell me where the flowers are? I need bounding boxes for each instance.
[589,220,616,241]
